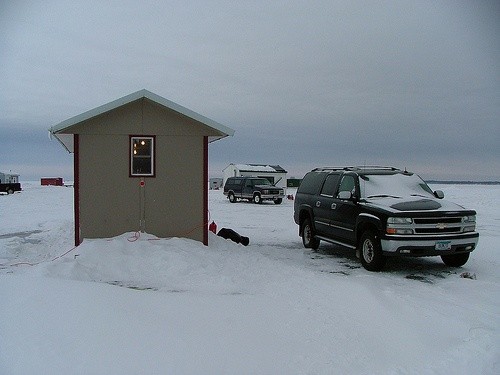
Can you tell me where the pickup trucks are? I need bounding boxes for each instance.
[0,178,22,195]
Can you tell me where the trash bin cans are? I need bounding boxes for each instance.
[287,194,293,200]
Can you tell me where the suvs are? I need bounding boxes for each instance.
[293,165,479,271]
[223,177,285,205]
